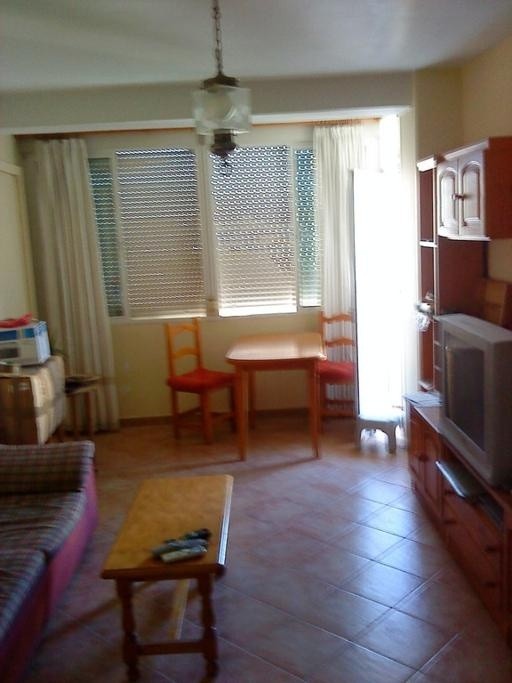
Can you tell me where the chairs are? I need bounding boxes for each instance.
[161,318,235,444]
[318,310,354,434]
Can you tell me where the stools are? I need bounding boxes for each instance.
[355,410,406,453]
[57,383,96,442]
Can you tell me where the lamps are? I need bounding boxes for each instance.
[190,0,252,158]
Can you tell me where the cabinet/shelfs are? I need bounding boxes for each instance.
[436,135,512,242]
[416,153,485,392]
[403,392,512,645]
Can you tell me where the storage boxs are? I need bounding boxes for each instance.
[1,355,68,445]
[0,321,52,367]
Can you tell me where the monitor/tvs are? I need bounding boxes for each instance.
[434,312,512,491]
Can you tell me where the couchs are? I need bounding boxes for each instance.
[0,439,99,683]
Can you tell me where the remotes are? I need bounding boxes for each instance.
[161,545,208,563]
[152,538,209,559]
[164,527,212,543]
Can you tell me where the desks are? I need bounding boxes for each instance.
[224,332,324,461]
